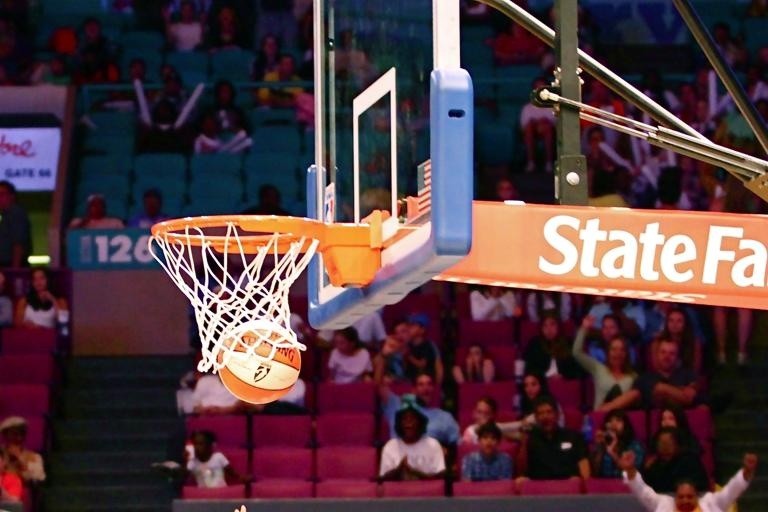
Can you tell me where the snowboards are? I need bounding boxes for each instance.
[217,320,303,406]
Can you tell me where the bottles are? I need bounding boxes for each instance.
[409,314,430,328]
[0,415,28,434]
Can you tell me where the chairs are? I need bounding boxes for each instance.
[66,1,768,356]
[1,2,78,502]
[715,349,752,368]
[176,330,716,502]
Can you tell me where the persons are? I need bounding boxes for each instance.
[15,264,69,330]
[3,417,46,486]
[4,181,33,268]
[185,430,246,487]
[178,254,312,414]
[28,0,429,230]
[317,1,768,224]
[326,287,768,511]
